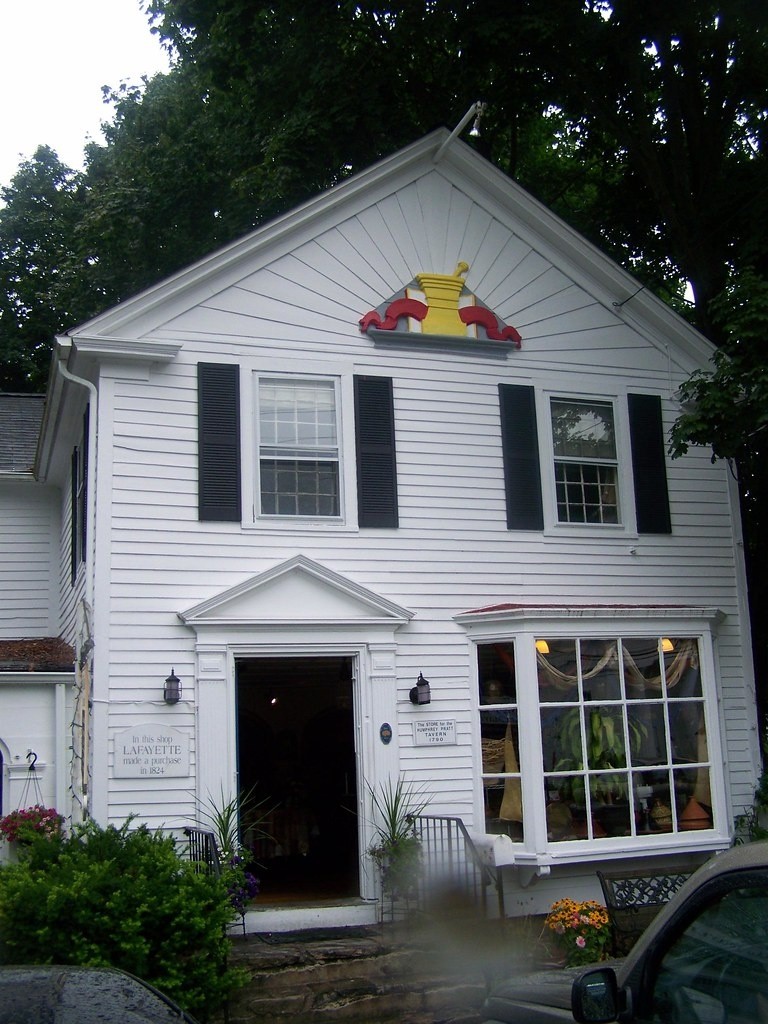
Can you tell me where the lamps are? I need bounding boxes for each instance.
[657,638,673,652]
[163,666,182,704]
[409,671,431,705]
[535,640,549,654]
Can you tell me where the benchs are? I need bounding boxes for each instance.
[593,861,702,957]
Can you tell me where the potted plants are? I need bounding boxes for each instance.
[502,895,548,977]
[554,708,648,839]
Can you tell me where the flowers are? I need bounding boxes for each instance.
[0,805,67,847]
[350,769,437,898]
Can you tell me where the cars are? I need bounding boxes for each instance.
[478,837,768,1024]
[0,966,204,1024]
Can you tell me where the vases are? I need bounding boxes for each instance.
[15,837,33,846]
[382,855,394,869]
